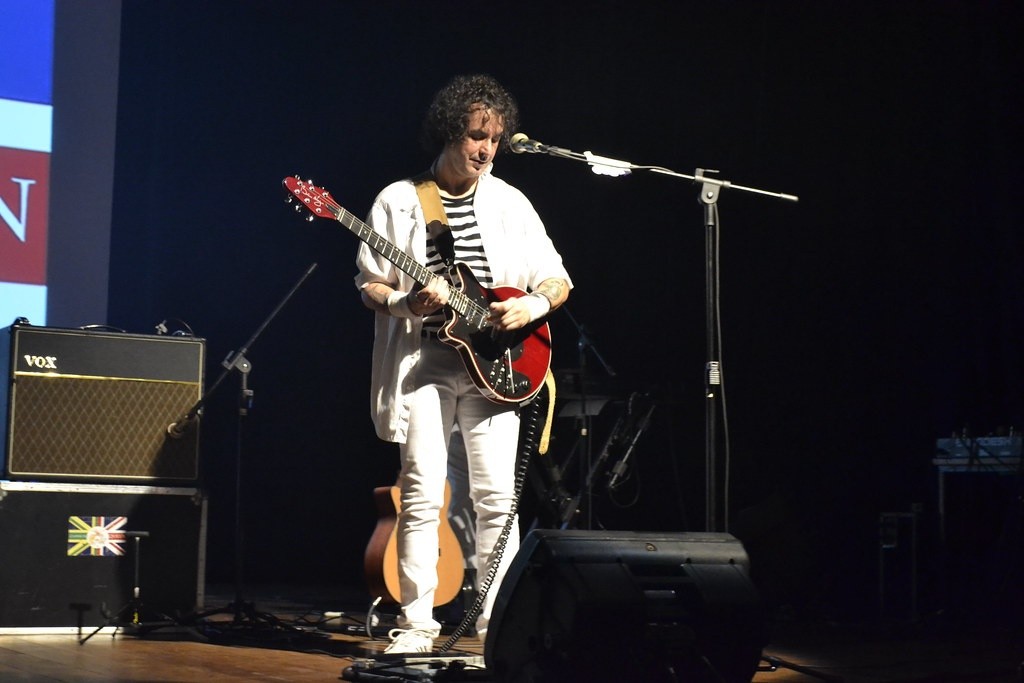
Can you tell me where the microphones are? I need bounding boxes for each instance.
[509,132,571,158]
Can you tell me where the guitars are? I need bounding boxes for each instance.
[281,172,555,405]
[361,462,466,610]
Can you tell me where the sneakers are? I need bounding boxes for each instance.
[383,632,437,655]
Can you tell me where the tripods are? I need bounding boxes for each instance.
[570,128,850,682]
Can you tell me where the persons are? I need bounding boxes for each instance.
[353,76,576,655]
